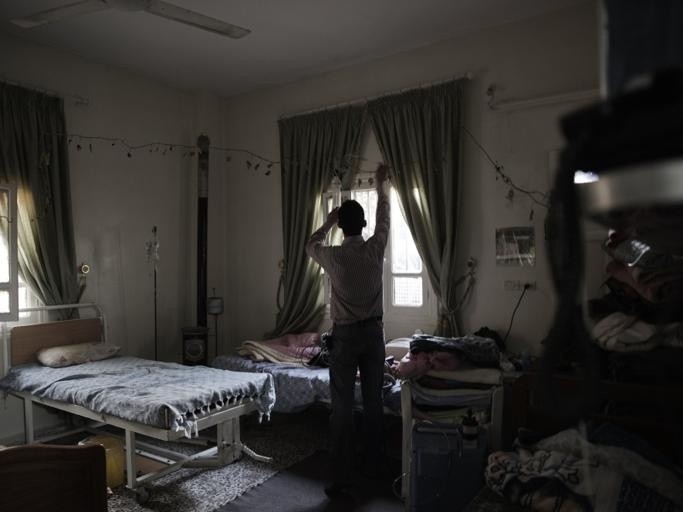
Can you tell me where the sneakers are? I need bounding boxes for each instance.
[324,475,378,499]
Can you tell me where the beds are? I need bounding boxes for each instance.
[210,351,399,446]
[1,304,277,491]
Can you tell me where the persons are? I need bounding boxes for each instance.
[304,162,401,497]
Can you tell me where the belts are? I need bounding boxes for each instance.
[333,315,383,331]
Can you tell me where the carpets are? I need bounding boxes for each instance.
[106,435,327,512]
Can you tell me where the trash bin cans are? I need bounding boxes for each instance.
[78,434,123,488]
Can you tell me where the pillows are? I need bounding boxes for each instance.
[34,340,124,369]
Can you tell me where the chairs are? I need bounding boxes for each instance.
[0,443,106,512]
[400,381,505,506]
